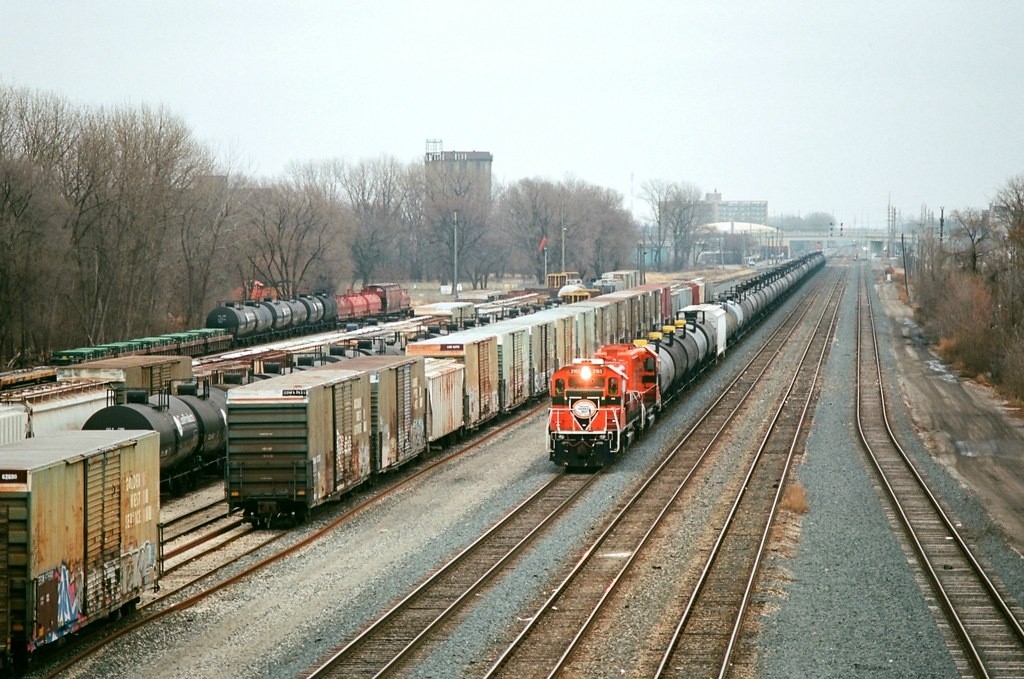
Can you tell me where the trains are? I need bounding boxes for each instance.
[81,288,601,498]
[546,250,827,474]
[0,281,414,392]
